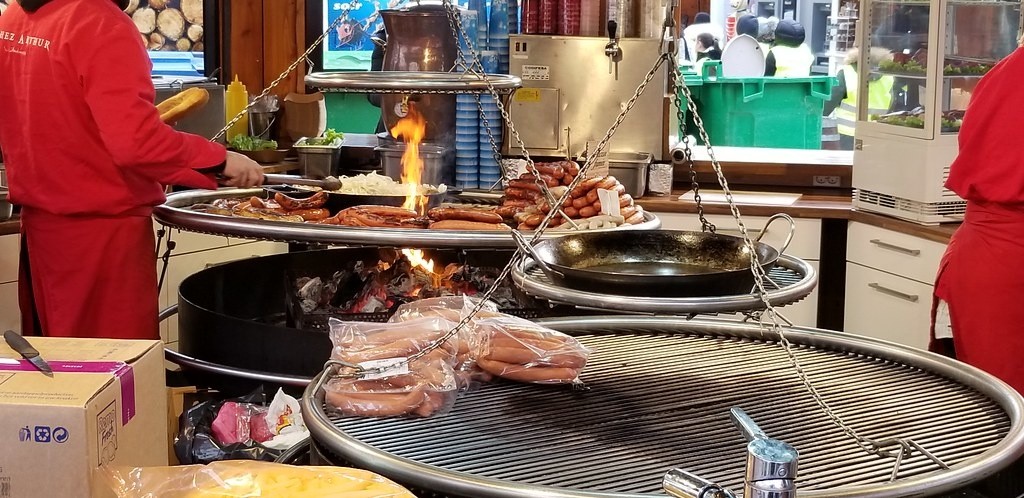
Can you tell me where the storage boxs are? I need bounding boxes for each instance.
[0,335,168,498]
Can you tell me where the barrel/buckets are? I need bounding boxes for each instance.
[249,109,281,142]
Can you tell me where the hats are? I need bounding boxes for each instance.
[756,16,779,37]
[694,12,710,23]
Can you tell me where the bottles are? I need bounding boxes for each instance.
[225,73,249,142]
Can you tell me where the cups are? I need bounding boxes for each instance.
[455,0,581,191]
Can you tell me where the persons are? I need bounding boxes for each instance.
[679,13,721,59]
[0,0,265,340]
[935,45,1024,396]
[736,15,771,56]
[764,19,815,78]
[822,33,894,150]
[694,33,722,76]
[367,29,386,134]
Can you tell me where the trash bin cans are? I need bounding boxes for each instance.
[676,59,840,151]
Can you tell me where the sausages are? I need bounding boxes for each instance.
[189,190,511,231]
[323,308,586,417]
[494,160,643,229]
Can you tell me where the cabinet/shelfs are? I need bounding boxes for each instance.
[633,189,964,351]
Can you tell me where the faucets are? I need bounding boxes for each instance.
[663,407,799,498]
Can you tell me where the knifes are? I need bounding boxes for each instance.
[3,330,53,379]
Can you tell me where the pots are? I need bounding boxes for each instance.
[281,183,447,214]
[511,213,796,298]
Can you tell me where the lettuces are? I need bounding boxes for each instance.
[299,127,344,148]
[232,132,276,151]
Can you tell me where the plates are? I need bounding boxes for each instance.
[228,148,290,164]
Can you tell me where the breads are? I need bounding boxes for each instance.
[155,87,210,124]
